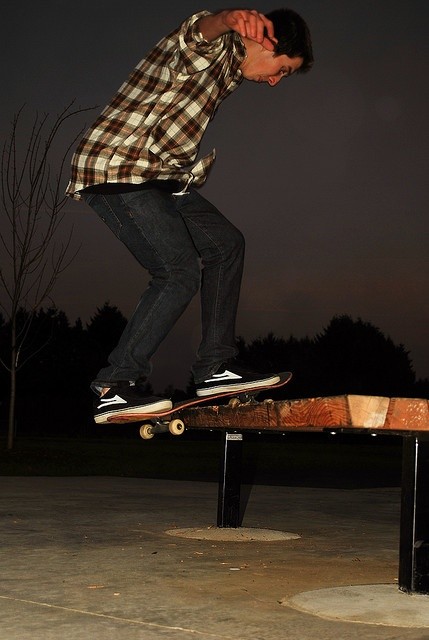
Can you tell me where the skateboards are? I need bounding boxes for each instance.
[106,371,294,440]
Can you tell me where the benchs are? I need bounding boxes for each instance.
[152,395,429,596]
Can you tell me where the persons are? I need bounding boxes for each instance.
[62,5,315,427]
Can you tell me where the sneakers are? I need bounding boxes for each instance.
[92,383,172,425]
[196,368,280,398]
